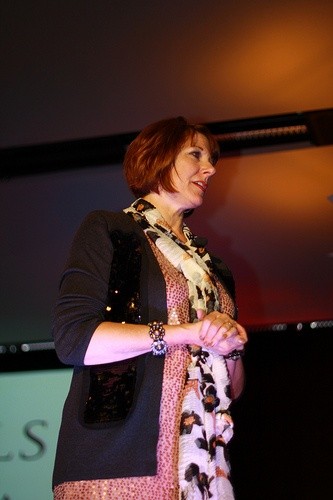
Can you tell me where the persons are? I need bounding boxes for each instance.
[53,118,247,500]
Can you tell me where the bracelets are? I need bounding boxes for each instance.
[149,321,167,355]
[224,351,246,361]
